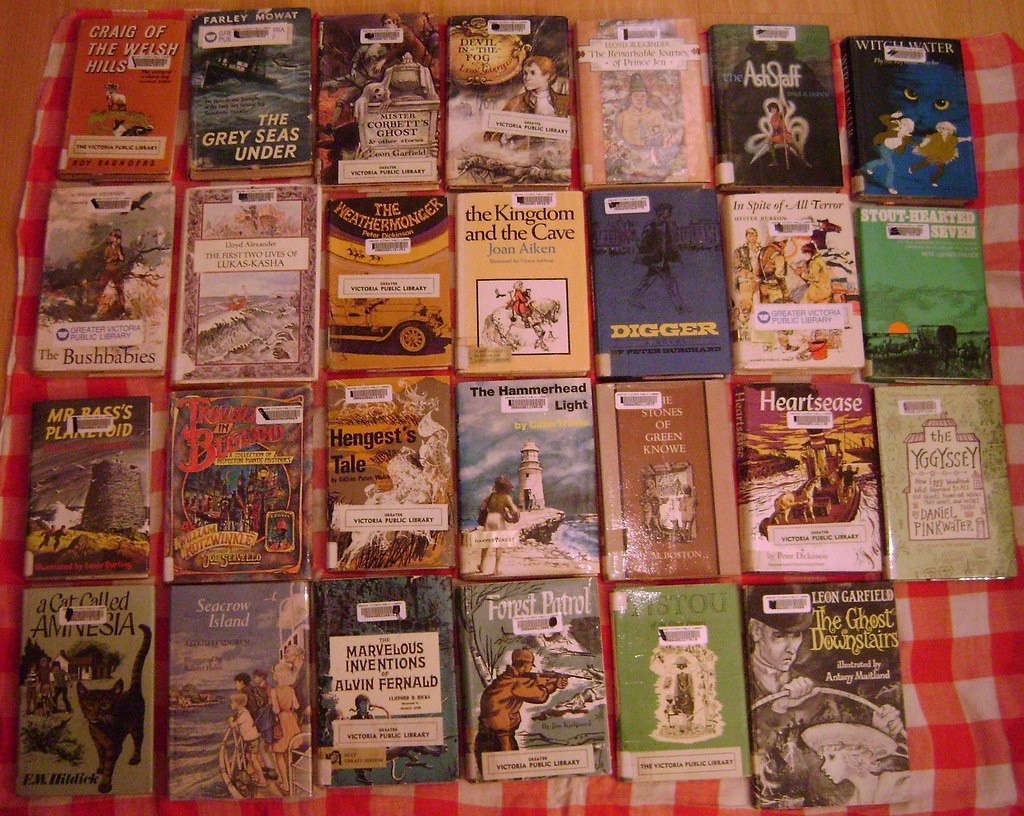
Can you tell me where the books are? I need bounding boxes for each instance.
[13,9,1015,795]
